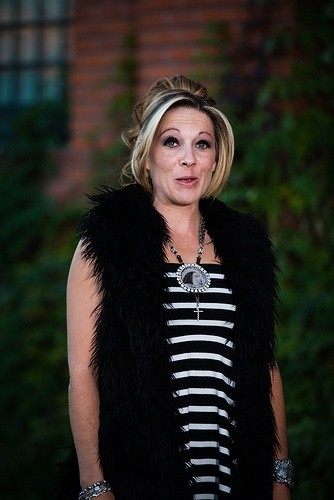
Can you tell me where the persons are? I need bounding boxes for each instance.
[68,74,293,500]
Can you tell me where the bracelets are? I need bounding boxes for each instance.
[79,476,114,500]
[271,459,293,487]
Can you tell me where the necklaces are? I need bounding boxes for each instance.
[166,215,211,322]
